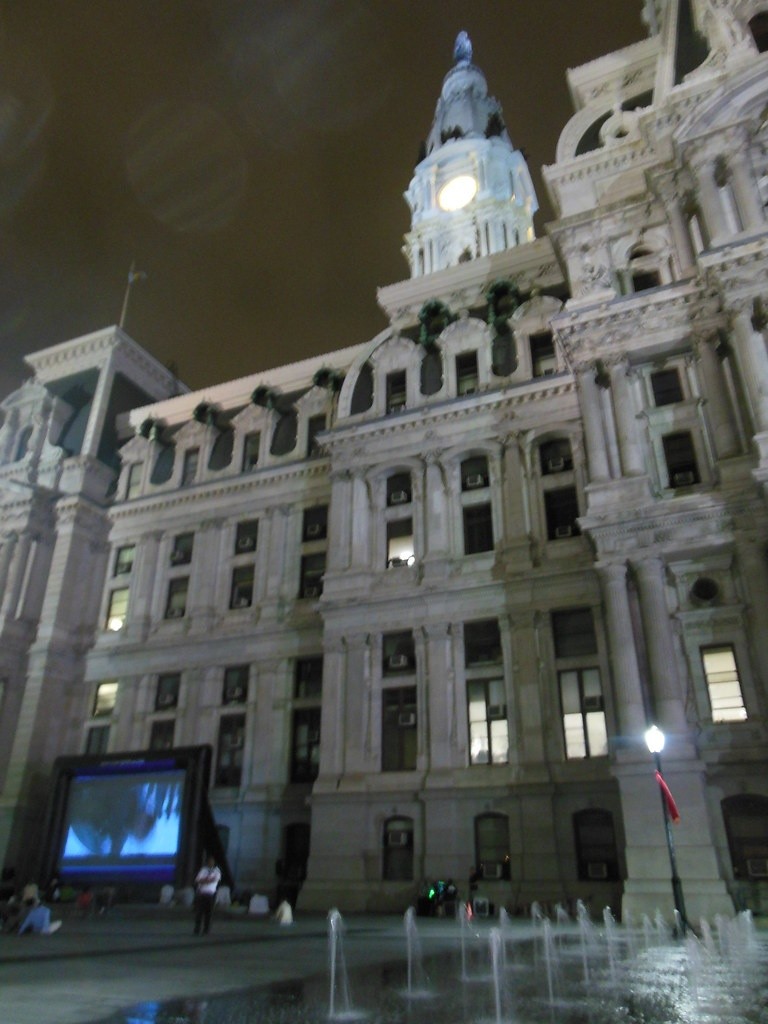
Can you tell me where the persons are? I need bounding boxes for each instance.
[0,878,115,936]
[194,856,221,937]
[416,866,480,918]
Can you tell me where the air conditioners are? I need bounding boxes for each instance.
[387,832,410,845]
[157,741,171,749]
[234,598,248,607]
[304,586,318,597]
[399,712,416,725]
[308,523,322,535]
[159,694,175,704]
[488,704,504,718]
[391,404,405,414]
[391,491,408,504]
[169,550,185,562]
[389,655,407,668]
[167,607,183,618]
[587,862,616,880]
[393,557,408,566]
[549,456,564,473]
[483,864,507,879]
[245,464,256,473]
[555,525,572,538]
[118,562,131,572]
[226,686,244,697]
[582,696,601,710]
[228,736,243,747]
[308,730,319,742]
[466,474,484,489]
[544,368,554,375]
[238,537,254,548]
[466,386,477,394]
[672,471,694,487]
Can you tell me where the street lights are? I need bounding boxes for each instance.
[643,720,690,943]
